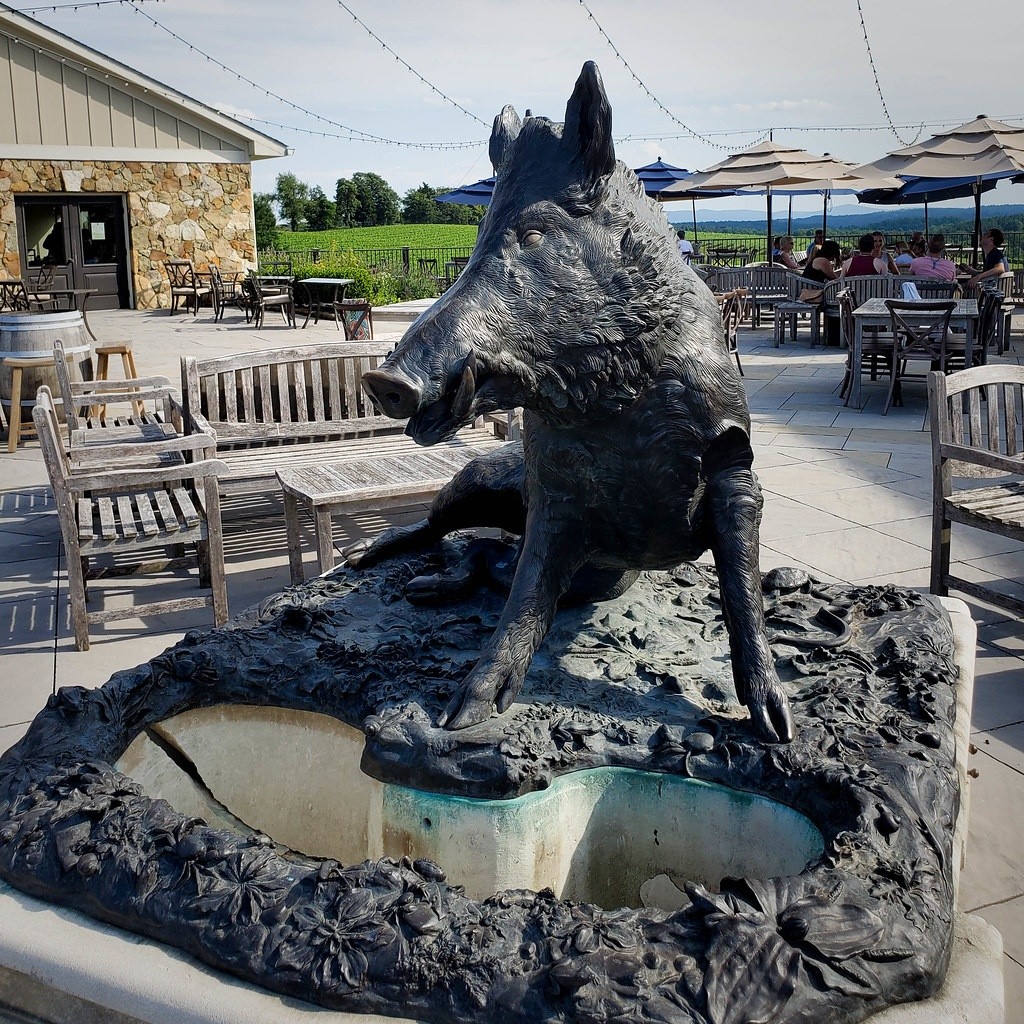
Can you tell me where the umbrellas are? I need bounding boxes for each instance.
[632,141,904,286]
[843,114,1024,273]
[432,176,497,206]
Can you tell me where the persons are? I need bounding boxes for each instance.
[677,231,694,266]
[772,229,1008,347]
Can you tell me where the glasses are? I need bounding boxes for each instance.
[788,242,793,245]
[817,236,821,239]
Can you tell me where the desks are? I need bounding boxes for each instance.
[70,423,186,495]
[445,261,467,291]
[298,277,355,331]
[943,247,1004,264]
[851,297,978,409]
[245,275,295,320]
[28,289,99,341]
[898,268,972,283]
[706,252,748,266]
[188,271,245,314]
[276,441,514,582]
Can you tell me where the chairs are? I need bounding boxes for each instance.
[682,243,1024,415]
[0,280,30,312]
[163,259,209,317]
[30,385,231,651]
[333,301,373,340]
[417,258,446,296]
[53,338,182,432]
[258,261,293,320]
[451,257,469,281]
[924,364,1024,619]
[27,265,58,310]
[247,267,296,330]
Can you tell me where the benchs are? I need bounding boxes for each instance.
[180,340,525,582]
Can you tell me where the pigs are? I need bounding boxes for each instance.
[338,57,794,743]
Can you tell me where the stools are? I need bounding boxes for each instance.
[3,351,74,453]
[94,339,146,417]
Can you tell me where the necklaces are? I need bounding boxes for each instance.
[862,254,869,255]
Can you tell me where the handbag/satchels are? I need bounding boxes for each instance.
[798,288,824,304]
